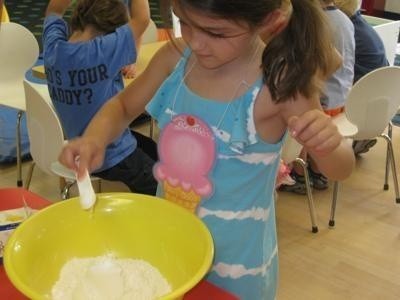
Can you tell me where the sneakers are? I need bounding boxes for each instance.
[279,170,313,195]
[309,169,329,190]
[352,139,378,156]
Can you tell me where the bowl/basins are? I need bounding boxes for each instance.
[3,191,215,300]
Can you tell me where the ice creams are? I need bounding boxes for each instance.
[154,115,215,215]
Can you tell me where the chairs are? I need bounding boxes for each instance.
[0,15,157,201]
[276,65,400,232]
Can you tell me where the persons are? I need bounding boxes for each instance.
[117,0,141,80]
[54,0,357,300]
[335,0,389,156]
[43,1,159,196]
[279,0,355,195]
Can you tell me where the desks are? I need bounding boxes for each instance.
[0,186,244,300]
[121,15,399,141]
